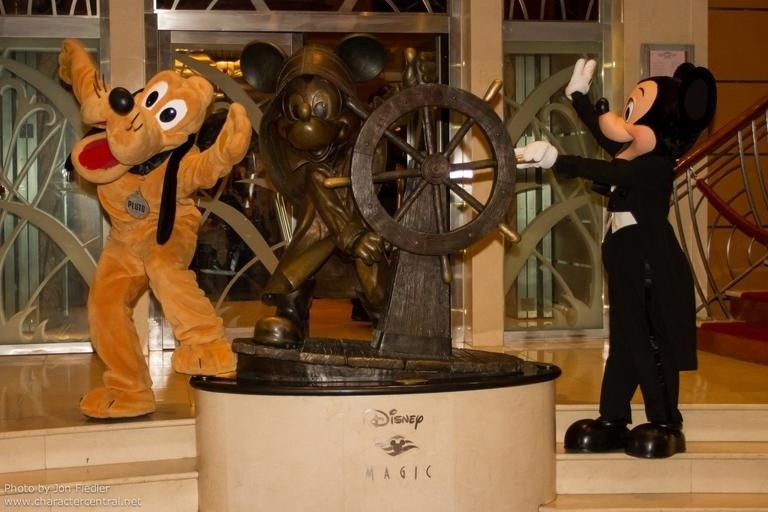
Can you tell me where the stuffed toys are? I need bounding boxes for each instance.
[58,37,253,419]
[514,59,717,459]
